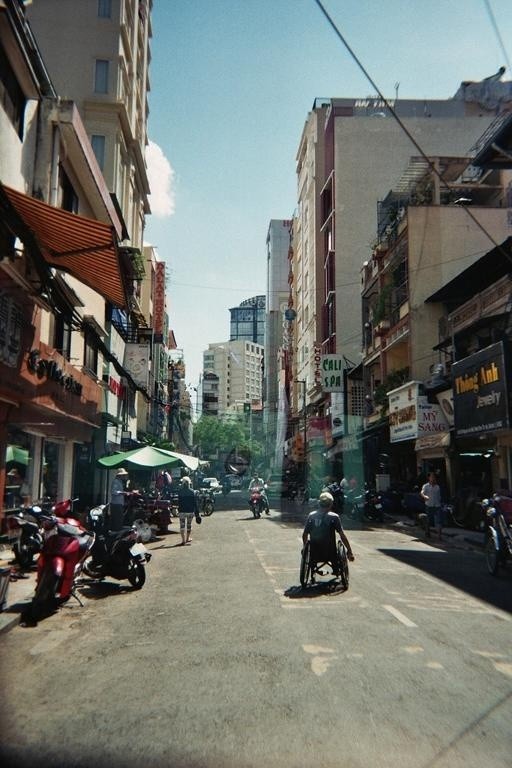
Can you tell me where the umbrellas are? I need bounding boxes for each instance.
[97,445,199,471]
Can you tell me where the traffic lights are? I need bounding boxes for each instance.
[244,404,250,412]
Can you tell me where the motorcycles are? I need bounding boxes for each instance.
[221,479,232,498]
[292,479,390,521]
[246,479,271,519]
[0,487,217,610]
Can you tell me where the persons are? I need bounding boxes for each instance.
[110,468,129,531]
[248,473,270,515]
[178,480,199,545]
[303,473,360,574]
[420,472,445,545]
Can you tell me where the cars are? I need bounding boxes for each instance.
[227,473,242,489]
[203,477,220,493]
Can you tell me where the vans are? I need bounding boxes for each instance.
[265,472,289,494]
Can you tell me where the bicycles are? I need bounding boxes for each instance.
[478,492,512,577]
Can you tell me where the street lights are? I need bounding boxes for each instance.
[294,379,307,479]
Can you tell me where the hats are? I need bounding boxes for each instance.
[319,492,334,505]
[117,469,127,475]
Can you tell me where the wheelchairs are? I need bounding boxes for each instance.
[295,519,352,591]
